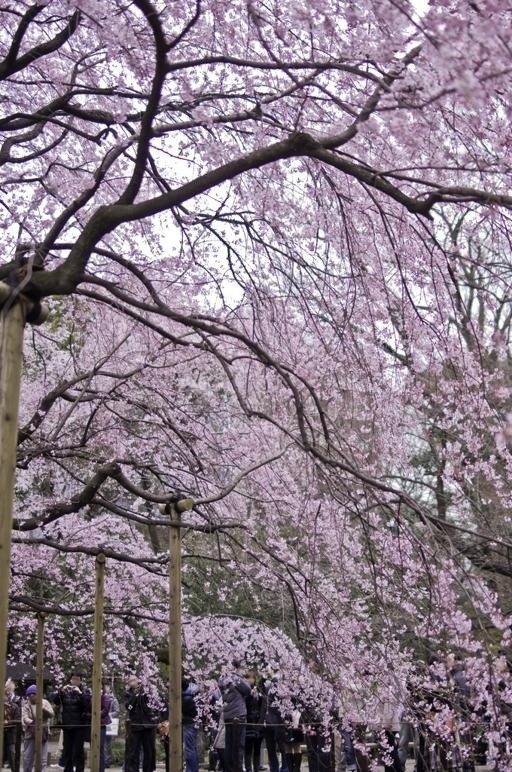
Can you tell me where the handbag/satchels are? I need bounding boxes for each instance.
[28,722,47,741]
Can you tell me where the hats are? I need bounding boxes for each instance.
[26,684,38,695]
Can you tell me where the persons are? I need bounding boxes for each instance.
[3,663,511,772]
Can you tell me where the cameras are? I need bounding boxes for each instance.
[67,686,72,691]
